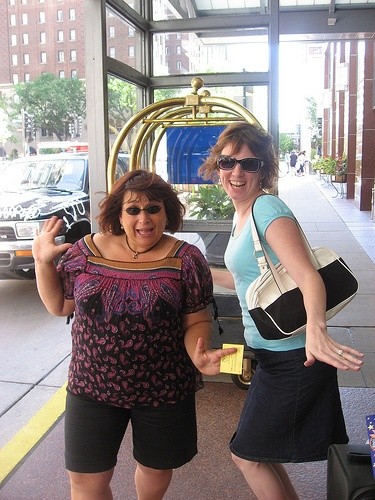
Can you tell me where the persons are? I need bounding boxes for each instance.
[197,123,365,500]
[31,169,237,500]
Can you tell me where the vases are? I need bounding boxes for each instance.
[335,174,347,183]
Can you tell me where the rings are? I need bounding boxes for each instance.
[338,348,345,355]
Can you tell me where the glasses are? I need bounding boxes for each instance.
[123,204,165,216]
[216,155,265,173]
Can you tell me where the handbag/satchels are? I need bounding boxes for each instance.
[245,194,359,340]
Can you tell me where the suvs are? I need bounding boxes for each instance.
[0,141,129,280]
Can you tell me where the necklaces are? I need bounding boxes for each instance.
[125,233,159,259]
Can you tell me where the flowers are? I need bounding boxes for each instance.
[313,154,352,176]
[185,182,235,221]
